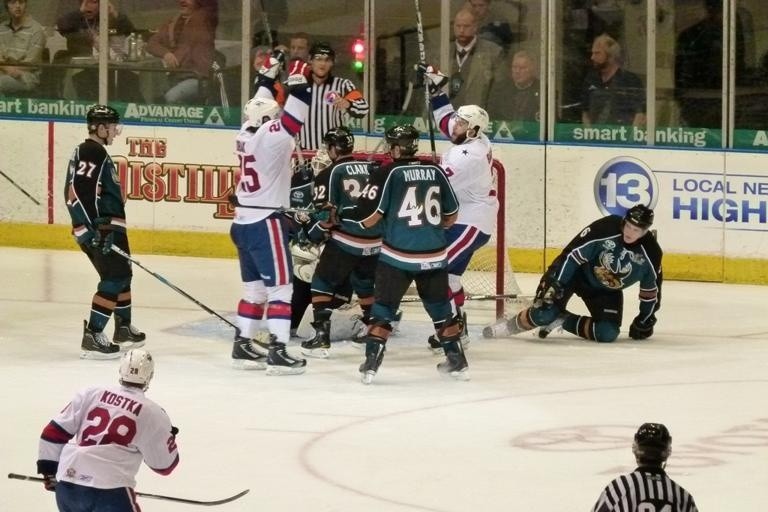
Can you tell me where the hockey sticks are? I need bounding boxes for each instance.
[9,473,249,506]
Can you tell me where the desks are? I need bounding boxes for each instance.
[1,59,202,104]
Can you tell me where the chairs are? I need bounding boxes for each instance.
[204,51,241,104]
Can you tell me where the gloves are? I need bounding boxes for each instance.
[317,200,338,226]
[94,226,113,254]
[629,316,657,340]
[43,474,59,492]
[265,50,288,64]
[424,63,450,91]
[533,281,557,310]
[290,62,311,82]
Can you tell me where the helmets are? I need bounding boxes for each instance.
[324,126,354,161]
[118,349,155,391]
[245,97,279,130]
[308,41,336,62]
[452,105,490,144]
[87,104,120,145]
[622,204,654,236]
[385,124,419,161]
[631,422,672,466]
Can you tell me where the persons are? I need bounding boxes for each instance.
[576,36,647,128]
[0,0,48,98]
[146,1,220,105]
[284,149,333,349]
[415,60,500,355]
[293,42,370,168]
[484,51,539,121]
[250,47,277,80]
[483,204,665,343]
[292,127,383,358]
[450,9,507,112]
[313,123,475,385]
[230,44,312,375]
[57,0,146,104]
[591,423,698,512]
[37,348,180,511]
[465,0,514,53]
[64,105,147,361]
[674,1,745,129]
[288,32,314,64]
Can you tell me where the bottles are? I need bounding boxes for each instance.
[136,34,144,61]
[127,32,138,61]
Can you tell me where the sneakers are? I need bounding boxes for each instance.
[359,341,386,372]
[350,317,370,343]
[437,339,468,374]
[539,309,561,339]
[231,336,268,363]
[268,343,306,368]
[300,321,332,347]
[81,319,120,353]
[112,324,146,343]
[483,321,512,338]
